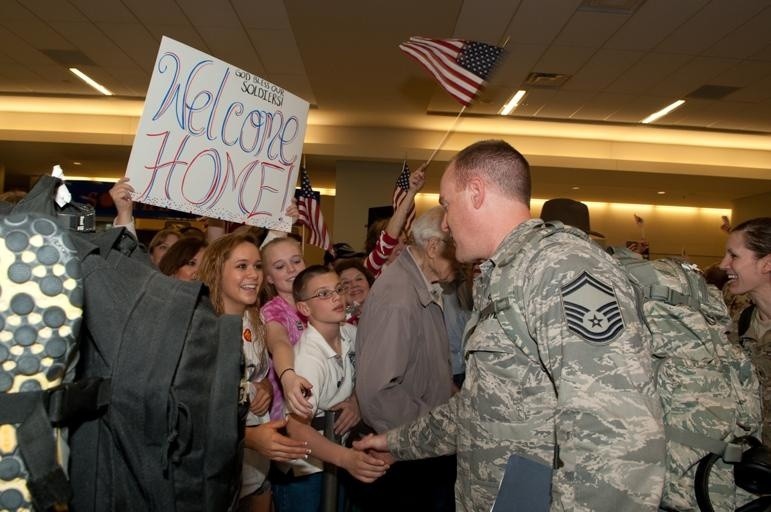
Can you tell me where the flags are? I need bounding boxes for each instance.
[398,33,503,108]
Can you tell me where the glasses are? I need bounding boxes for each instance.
[300,282,346,302]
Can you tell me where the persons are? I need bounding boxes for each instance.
[2,136,771,511]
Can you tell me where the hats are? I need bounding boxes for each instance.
[539,198,607,241]
